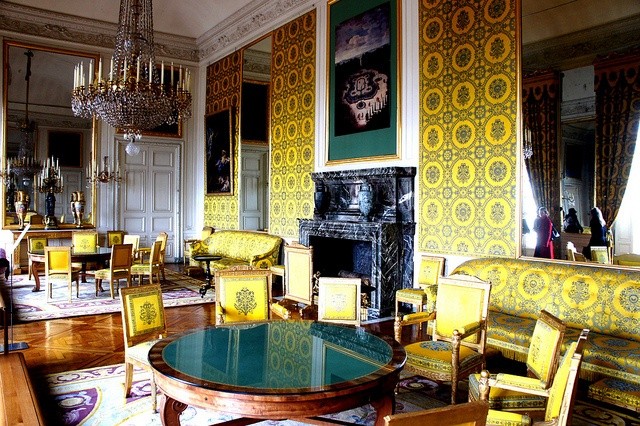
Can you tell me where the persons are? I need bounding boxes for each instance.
[533,207,555,259]
[562,208,584,234]
[215,149,230,192]
[588,207,607,260]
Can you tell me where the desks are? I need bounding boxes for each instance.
[27,243,113,292]
[192,256,223,298]
[3,228,97,277]
[148,318,407,426]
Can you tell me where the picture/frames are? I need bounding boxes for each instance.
[324,0,403,166]
[44,127,85,171]
[203,105,234,196]
[111,58,186,139]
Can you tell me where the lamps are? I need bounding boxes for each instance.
[70,0,192,158]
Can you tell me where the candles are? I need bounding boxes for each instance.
[188,69,191,92]
[184,68,189,92]
[31,157,34,167]
[17,155,20,164]
[27,157,29,170]
[14,157,17,165]
[176,80,180,95]
[37,174,40,187]
[523,126,529,148]
[136,56,140,83]
[43,158,47,168]
[88,59,94,85]
[80,61,84,86]
[22,156,26,165]
[160,58,165,86]
[61,173,64,188]
[46,168,49,179]
[98,57,102,82]
[178,64,184,88]
[123,55,127,82]
[170,60,174,87]
[528,126,532,146]
[51,156,54,169]
[72,65,76,89]
[47,157,50,168]
[40,177,43,188]
[7,157,11,173]
[148,57,152,84]
[76,62,80,87]
[56,157,59,172]
[110,55,114,81]
[11,156,13,164]
[58,166,61,181]
[42,169,45,178]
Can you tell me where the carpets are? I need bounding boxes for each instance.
[33,356,469,426]
[3,268,216,324]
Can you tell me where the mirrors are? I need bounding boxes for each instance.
[3,40,100,239]
[516,0,639,274]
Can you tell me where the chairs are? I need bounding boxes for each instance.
[486,329,590,426]
[184,225,213,265]
[124,234,139,264]
[394,273,492,404]
[470,311,565,422]
[95,243,134,300]
[564,240,575,259]
[580,225,592,234]
[613,252,639,266]
[394,256,446,341]
[384,401,488,426]
[72,230,105,292]
[45,245,83,306]
[214,270,289,325]
[141,232,169,283]
[27,235,49,281]
[107,230,123,247]
[118,287,167,414]
[576,252,585,260]
[590,244,610,265]
[130,241,162,285]
[318,278,362,328]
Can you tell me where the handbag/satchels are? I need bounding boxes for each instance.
[551,224,560,241]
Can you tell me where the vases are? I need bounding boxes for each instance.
[12,190,31,227]
[70,191,85,225]
[70,192,78,224]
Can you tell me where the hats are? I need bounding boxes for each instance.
[569,208,577,214]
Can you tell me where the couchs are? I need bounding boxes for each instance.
[190,229,283,287]
[453,257,639,418]
